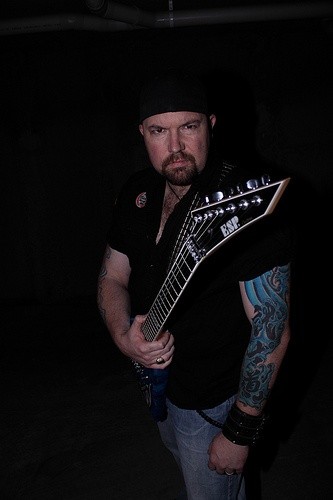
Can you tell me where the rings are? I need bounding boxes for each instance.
[226,471,234,476]
[155,358,165,364]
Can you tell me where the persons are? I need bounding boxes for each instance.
[97,75,291,500]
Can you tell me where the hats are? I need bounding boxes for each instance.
[139,75,209,124]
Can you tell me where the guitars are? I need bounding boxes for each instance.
[119,174,292,426]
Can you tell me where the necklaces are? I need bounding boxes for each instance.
[167,181,180,201]
